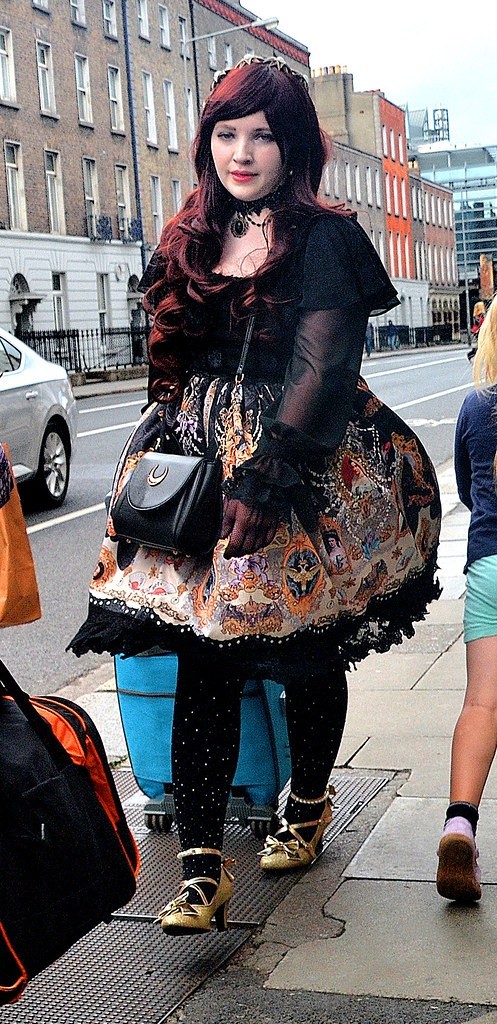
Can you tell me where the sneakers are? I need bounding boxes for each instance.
[435,815,482,900]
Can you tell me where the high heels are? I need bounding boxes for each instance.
[158,847,236,935]
[257,785,336,871]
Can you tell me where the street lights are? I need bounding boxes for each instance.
[182,16,279,190]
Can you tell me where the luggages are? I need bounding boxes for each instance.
[113,652,292,836]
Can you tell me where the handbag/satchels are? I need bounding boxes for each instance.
[0,442,42,629]
[105,451,224,558]
[0,657,141,1007]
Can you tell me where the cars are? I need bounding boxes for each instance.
[0,329,77,510]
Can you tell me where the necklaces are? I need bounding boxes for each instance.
[231,206,263,236]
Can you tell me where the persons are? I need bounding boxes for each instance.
[384,320,399,351]
[436,294,497,903]
[364,322,374,357]
[63,52,444,934]
[467,301,486,362]
